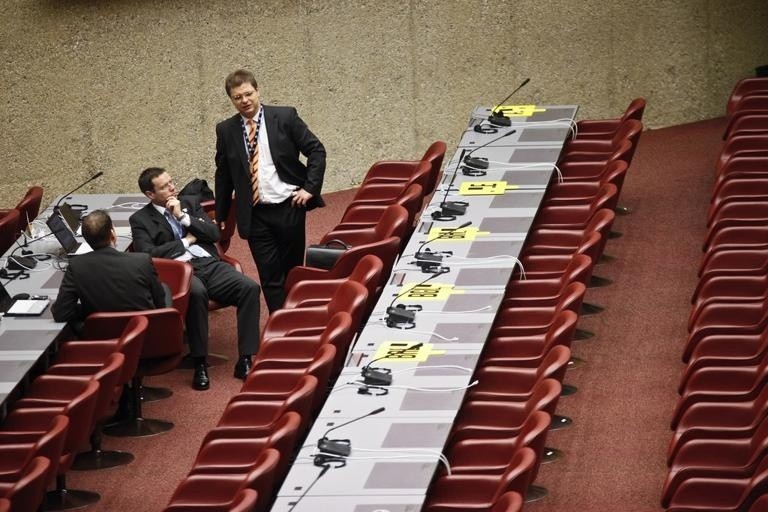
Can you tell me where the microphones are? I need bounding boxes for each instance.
[386,270,445,323]
[8,227,64,270]
[463,130,516,169]
[488,78,530,126]
[53,171,103,221]
[364,343,423,385]
[318,406,385,457]
[288,464,330,512]
[415,221,472,266]
[440,149,466,215]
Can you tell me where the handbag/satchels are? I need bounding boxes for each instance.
[306,239,352,269]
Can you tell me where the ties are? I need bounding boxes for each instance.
[247,119,259,207]
[164,209,184,240]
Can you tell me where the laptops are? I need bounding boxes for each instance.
[0,281,50,317]
[46,209,94,258]
[60,202,82,236]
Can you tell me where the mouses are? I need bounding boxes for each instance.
[13,293,30,300]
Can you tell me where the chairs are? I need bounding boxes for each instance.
[0,177,239,511]
[164,141,445,512]
[662,61,768,511]
[430,96,639,511]
[1,186,43,253]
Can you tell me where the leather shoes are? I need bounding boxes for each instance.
[194,365,209,390]
[234,359,251,379]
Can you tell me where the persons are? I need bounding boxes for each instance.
[49,210,167,425]
[213,69,325,317]
[129,167,262,393]
